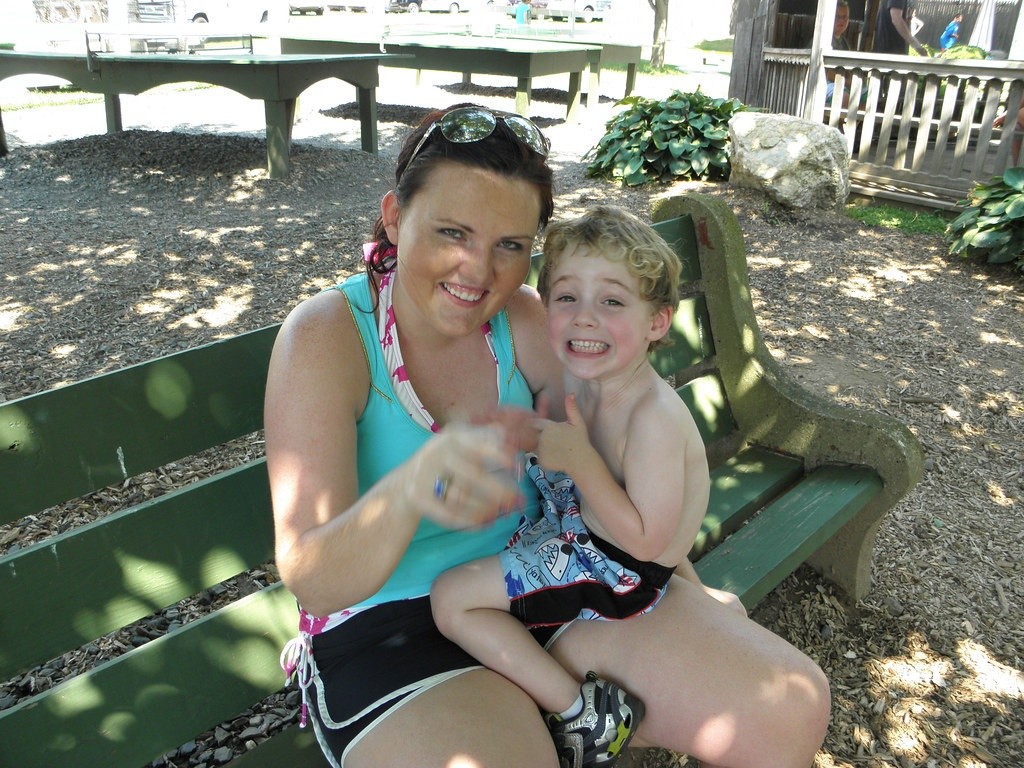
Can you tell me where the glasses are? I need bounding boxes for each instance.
[836,14,849,20]
[398,107,551,188]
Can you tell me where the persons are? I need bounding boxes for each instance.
[871,0,928,93]
[264,105,832,768]
[430,212,710,768]
[993,79,1024,167]
[807,1,868,134]
[940,13,963,51]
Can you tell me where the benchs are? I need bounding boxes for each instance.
[0,189,926,768]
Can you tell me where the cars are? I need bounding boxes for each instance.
[174,1,609,45]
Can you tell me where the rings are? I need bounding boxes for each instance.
[434,476,450,502]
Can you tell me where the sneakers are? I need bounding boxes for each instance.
[544,670,645,768]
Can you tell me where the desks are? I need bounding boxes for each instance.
[281,28,659,121]
[0,49,415,179]
